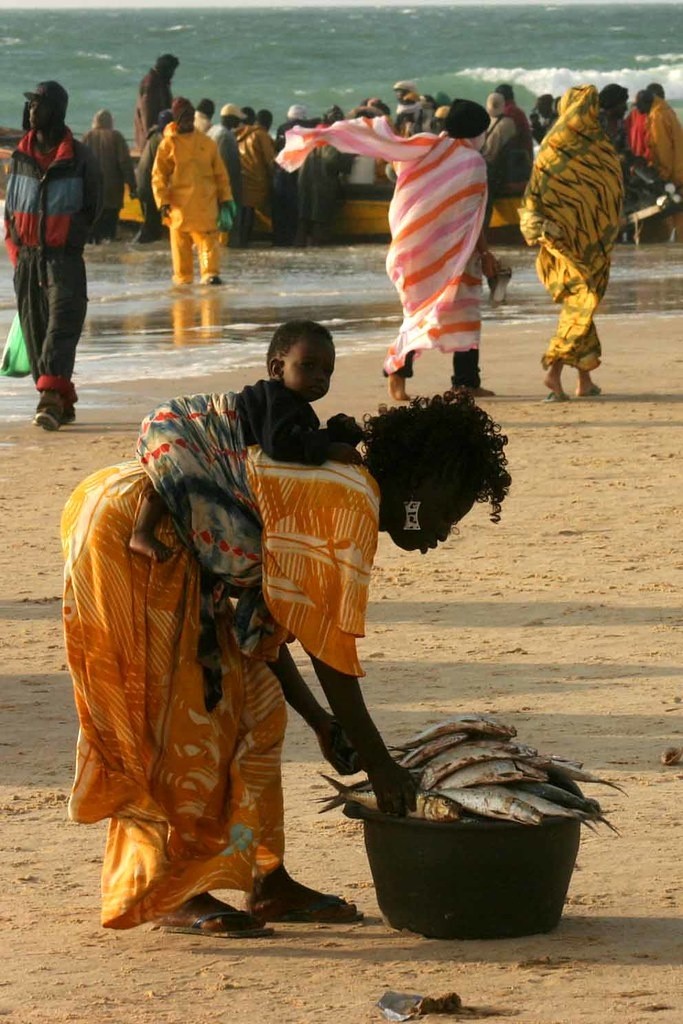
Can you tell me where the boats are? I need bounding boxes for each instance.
[0,123,680,241]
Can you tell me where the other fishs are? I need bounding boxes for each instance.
[1,347,31,378]
[315,717,630,838]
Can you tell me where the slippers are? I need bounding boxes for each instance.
[542,391,567,403]
[263,894,364,922]
[160,910,274,938]
[577,385,600,396]
[490,258,512,307]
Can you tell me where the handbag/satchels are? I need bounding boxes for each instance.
[0,311,32,377]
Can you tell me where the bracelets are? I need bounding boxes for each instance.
[476,248,492,258]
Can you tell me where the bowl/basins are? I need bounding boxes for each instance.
[343,801,583,939]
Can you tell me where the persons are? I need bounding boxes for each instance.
[599,83,683,241]
[275,99,499,399]
[193,79,451,248]
[481,83,560,183]
[60,387,511,938]
[79,109,138,243]
[517,85,624,403]
[133,54,180,153]
[129,319,369,563]
[133,110,176,243]
[151,96,236,286]
[4,81,105,431]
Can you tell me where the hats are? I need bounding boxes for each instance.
[220,103,249,120]
[394,80,415,93]
[173,97,194,123]
[92,110,114,128]
[21,81,69,140]
[287,105,307,121]
[434,107,450,118]
[447,99,491,138]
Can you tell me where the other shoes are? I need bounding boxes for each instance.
[33,404,63,431]
[32,406,76,425]
